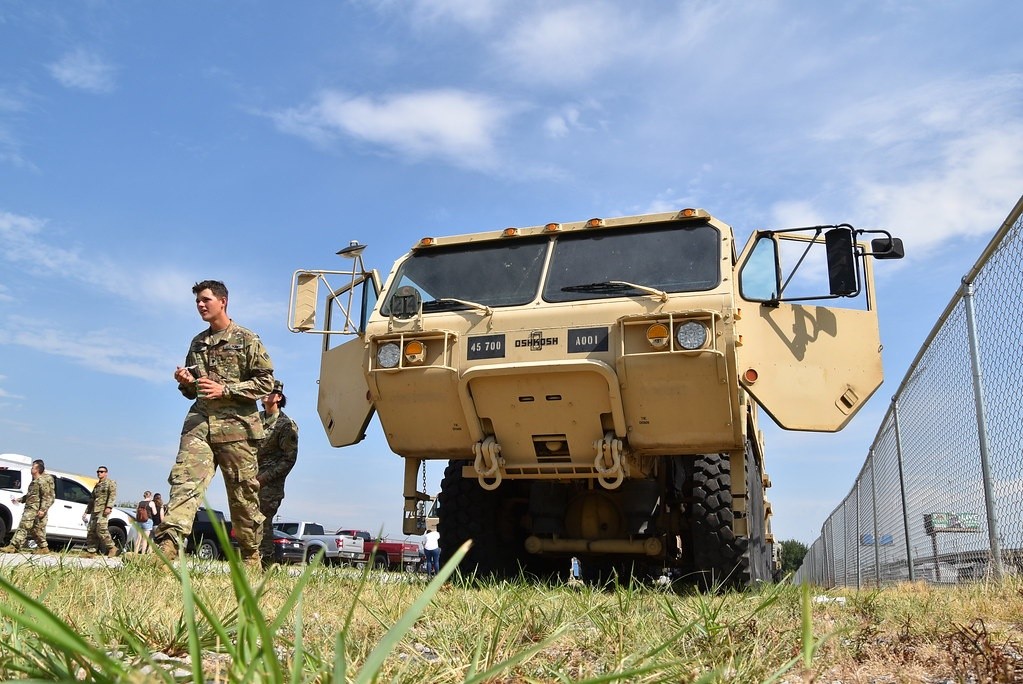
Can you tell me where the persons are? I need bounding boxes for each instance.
[33,459,56,537]
[80,466,119,559]
[122,279,275,574]
[422,529,442,578]
[0,463,50,555]
[148,493,165,553]
[133,491,157,555]
[255,380,299,570]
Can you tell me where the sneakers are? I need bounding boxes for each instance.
[241,555,262,580]
[122,538,178,568]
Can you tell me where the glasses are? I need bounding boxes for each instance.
[97,471,106,473]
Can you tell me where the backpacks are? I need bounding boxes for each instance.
[137,503,148,522]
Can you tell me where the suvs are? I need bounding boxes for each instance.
[0,459,140,558]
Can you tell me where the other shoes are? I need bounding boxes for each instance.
[108,546,119,558]
[79,552,95,559]
[0,544,16,553]
[31,547,49,554]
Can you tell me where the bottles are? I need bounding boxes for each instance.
[83,516,91,528]
[10,494,18,507]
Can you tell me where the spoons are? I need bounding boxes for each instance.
[180,365,198,370]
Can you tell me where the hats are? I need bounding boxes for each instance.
[273,379,283,392]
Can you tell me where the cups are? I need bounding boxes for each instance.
[194,378,209,398]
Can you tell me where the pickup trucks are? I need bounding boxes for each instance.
[334,529,422,572]
[272,520,366,567]
[185,508,241,560]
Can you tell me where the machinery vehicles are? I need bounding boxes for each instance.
[285,205,905,592]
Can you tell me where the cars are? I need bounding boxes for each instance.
[260,530,305,567]
